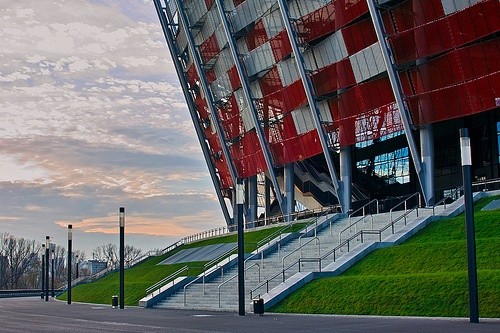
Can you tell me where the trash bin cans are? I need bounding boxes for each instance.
[112,295,119,309]
[40,292,45,301]
[252,298,265,316]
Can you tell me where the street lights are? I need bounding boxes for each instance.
[67,223,72,304]
[118,205,126,309]
[46,235,49,301]
[459,125,480,323]
[75,255,79,278]
[40,243,45,299]
[235,176,246,316]
[51,250,55,296]
